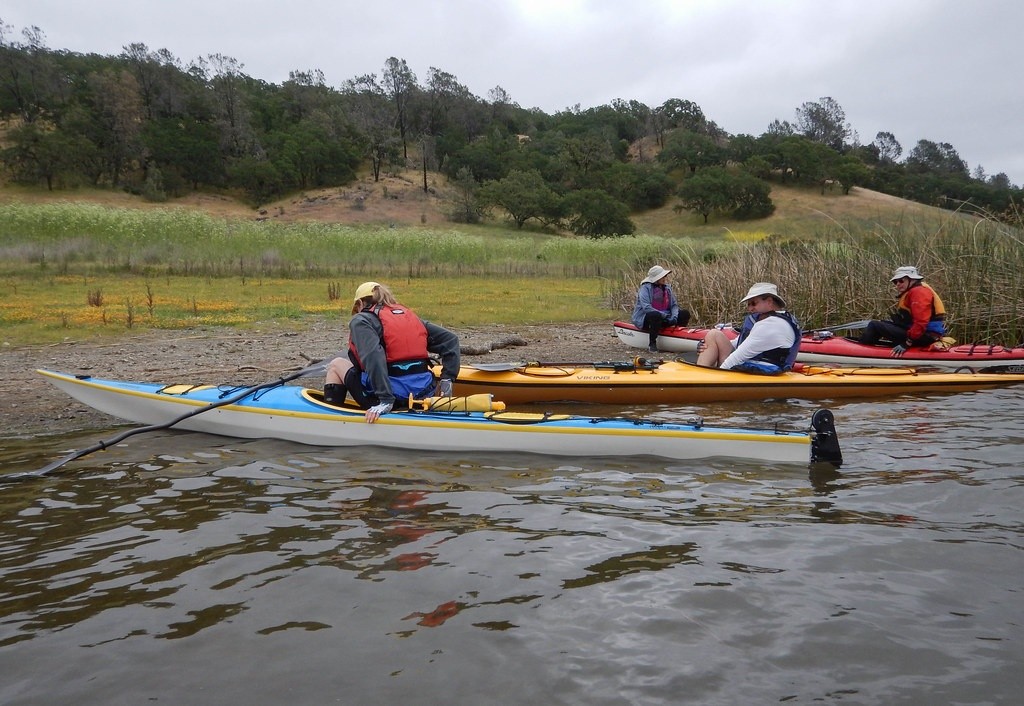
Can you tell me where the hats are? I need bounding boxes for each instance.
[739,282,786,308]
[641,265,672,283]
[890,266,924,282]
[351,281,380,315]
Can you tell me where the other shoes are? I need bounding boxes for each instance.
[648,343,658,354]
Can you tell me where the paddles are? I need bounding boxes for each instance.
[800,319,873,334]
[468,359,634,372]
[0,348,352,481]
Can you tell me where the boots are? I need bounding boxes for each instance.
[324,384,348,407]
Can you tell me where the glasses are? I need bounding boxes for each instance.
[893,279,904,283]
[748,296,766,308]
[665,274,667,277]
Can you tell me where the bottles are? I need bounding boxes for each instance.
[715,324,732,329]
[633,357,646,366]
[814,331,833,337]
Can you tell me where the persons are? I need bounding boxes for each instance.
[860,267,945,358]
[632,265,690,354]
[324,282,460,424]
[696,282,802,375]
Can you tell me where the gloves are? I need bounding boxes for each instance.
[672,317,677,324]
[664,317,674,323]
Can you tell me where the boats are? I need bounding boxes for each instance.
[33,366,846,466]
[419,360,1024,406]
[612,318,1024,374]
